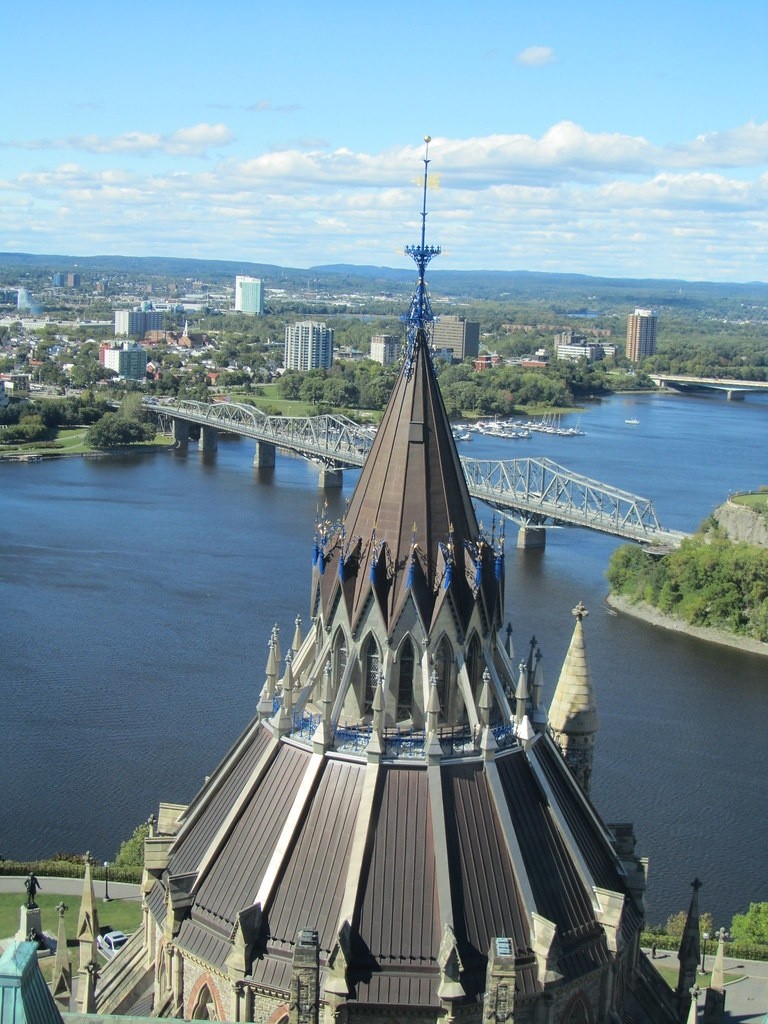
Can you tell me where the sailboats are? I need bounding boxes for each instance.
[530,406,587,438]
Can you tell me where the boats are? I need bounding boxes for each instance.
[446,413,533,441]
[625,418,640,425]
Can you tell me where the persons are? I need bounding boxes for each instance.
[29,928,44,949]
[651,942,656,959]
[24,872,43,909]
